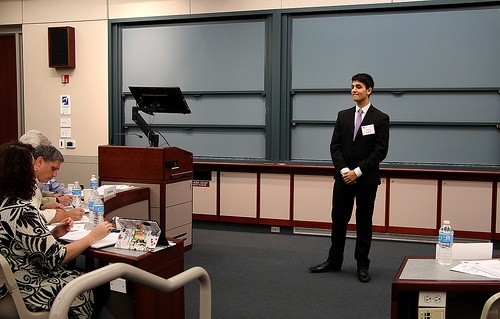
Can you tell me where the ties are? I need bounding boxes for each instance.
[354,109,363,139]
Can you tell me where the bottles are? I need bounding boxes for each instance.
[72,181,81,206]
[437,220,454,266]
[89,174,98,190]
[87,190,104,227]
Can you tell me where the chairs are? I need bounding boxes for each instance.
[0,254,50,319]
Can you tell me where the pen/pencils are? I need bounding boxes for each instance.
[72,204,76,208]
[108,216,115,223]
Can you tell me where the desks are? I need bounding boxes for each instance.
[55,224,185,319]
[390,256,500,319]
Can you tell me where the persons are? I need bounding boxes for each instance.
[309,73,389,282]
[0,140,114,319]
[31,145,85,225]
[19,130,73,195]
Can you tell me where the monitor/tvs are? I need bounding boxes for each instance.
[128,86,190,115]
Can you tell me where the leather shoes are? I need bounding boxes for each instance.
[357,261,371,283]
[309,261,341,272]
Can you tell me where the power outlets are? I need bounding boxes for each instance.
[418,308,445,319]
[418,291,446,307]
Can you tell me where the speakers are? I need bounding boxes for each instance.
[47,27,75,67]
